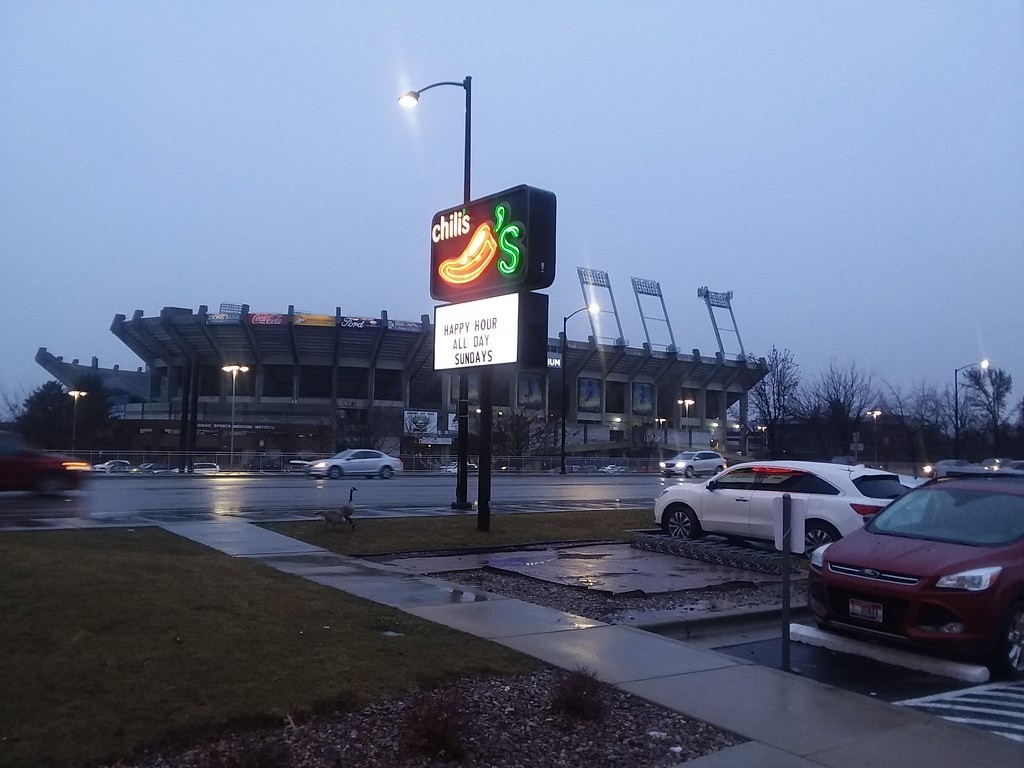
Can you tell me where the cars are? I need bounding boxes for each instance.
[0,431,91,495]
[652,458,914,561]
[552,464,630,473]
[91,460,222,475]
[306,449,405,479]
[440,461,519,472]
[660,449,728,478]
[807,459,1024,680]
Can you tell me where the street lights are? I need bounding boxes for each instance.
[561,305,601,473]
[654,418,667,459]
[678,398,694,448]
[223,364,250,469]
[68,390,87,459]
[954,358,991,458]
[396,76,496,532]
[865,410,882,468]
